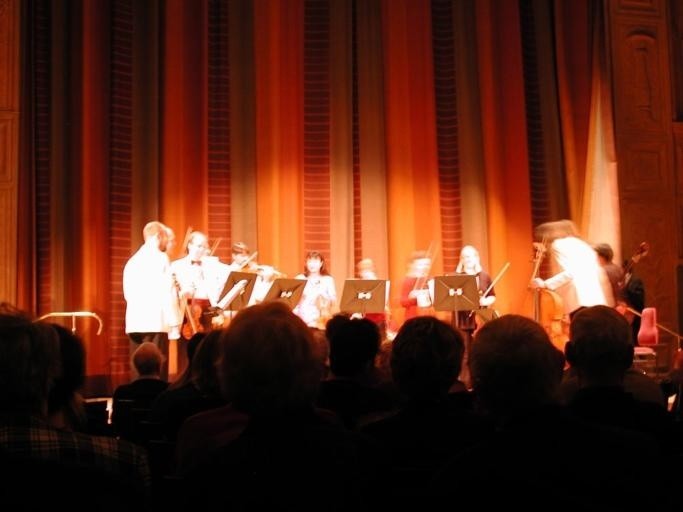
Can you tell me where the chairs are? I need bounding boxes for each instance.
[631,307,659,380]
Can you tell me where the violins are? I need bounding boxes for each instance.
[183,282,205,341]
[475,283,500,334]
[240,258,288,279]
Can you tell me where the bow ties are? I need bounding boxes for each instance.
[191,259,203,267]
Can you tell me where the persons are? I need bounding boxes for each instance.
[450,245,495,389]
[171,304,347,501]
[595,245,618,308]
[176,335,220,407]
[227,244,258,288]
[621,260,644,348]
[352,257,390,325]
[124,220,179,381]
[2,311,155,505]
[321,315,386,413]
[528,221,612,321]
[42,322,83,431]
[454,314,618,507]
[287,252,337,348]
[171,230,218,344]
[372,320,484,508]
[102,340,172,448]
[571,306,680,508]
[402,251,436,320]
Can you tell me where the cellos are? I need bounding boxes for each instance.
[520,241,569,355]
[610,239,651,295]
